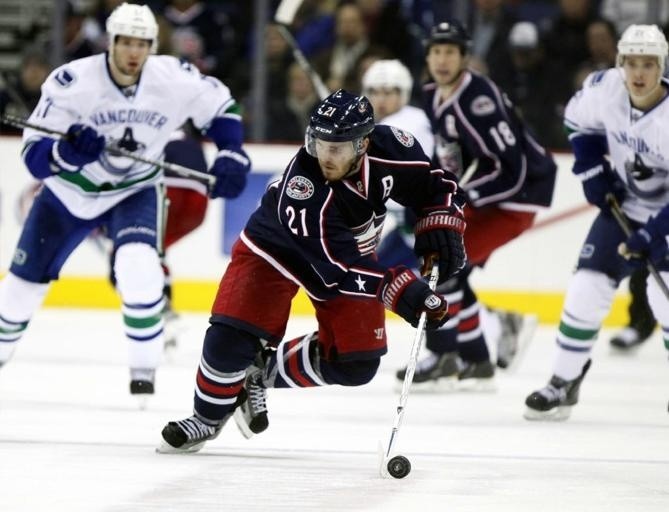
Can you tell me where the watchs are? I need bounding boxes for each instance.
[579,66,657,350]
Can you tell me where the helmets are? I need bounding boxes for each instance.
[364,59,414,103]
[106,3,158,41]
[423,22,469,56]
[616,23,667,70]
[310,88,375,143]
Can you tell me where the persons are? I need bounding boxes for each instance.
[1,1,253,399]
[263,1,669,151]
[151,86,467,454]
[361,58,539,385]
[1,1,253,144]
[518,21,669,414]
[417,20,559,373]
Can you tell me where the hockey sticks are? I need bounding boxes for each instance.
[275,0,333,102]
[380,264,439,481]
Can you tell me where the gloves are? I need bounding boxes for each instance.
[573,157,627,210]
[51,125,105,174]
[210,147,250,199]
[617,216,662,267]
[413,204,468,284]
[377,265,448,328]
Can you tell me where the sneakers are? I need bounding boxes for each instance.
[162,414,217,448]
[240,352,268,434]
[131,367,155,393]
[610,316,656,348]
[397,311,522,382]
[526,361,591,410]
